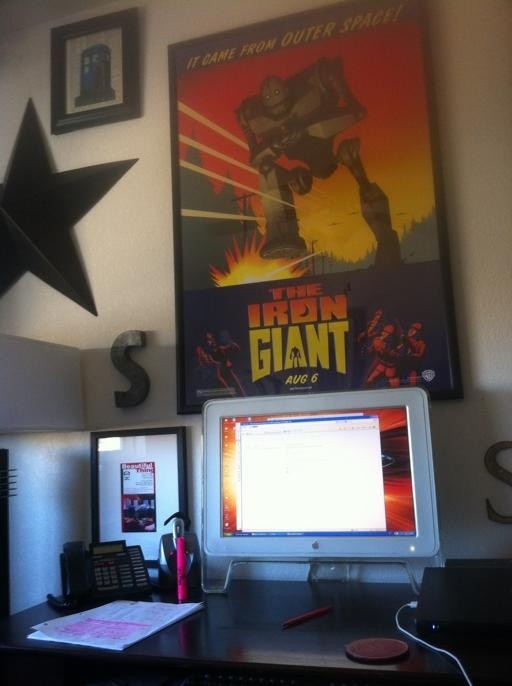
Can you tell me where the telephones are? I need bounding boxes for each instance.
[60,540,152,607]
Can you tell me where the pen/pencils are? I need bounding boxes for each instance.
[281,604,333,628]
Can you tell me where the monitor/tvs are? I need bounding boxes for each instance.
[200,387,445,596]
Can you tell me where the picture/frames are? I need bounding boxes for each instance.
[90,426,189,585]
[50,7,142,135]
[165,0,462,415]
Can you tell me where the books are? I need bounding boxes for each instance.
[26,598,205,650]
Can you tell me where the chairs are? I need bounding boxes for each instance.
[0,581,512,686]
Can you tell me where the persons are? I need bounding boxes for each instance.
[355,309,428,390]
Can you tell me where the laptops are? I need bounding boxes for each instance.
[414,564,512,652]
